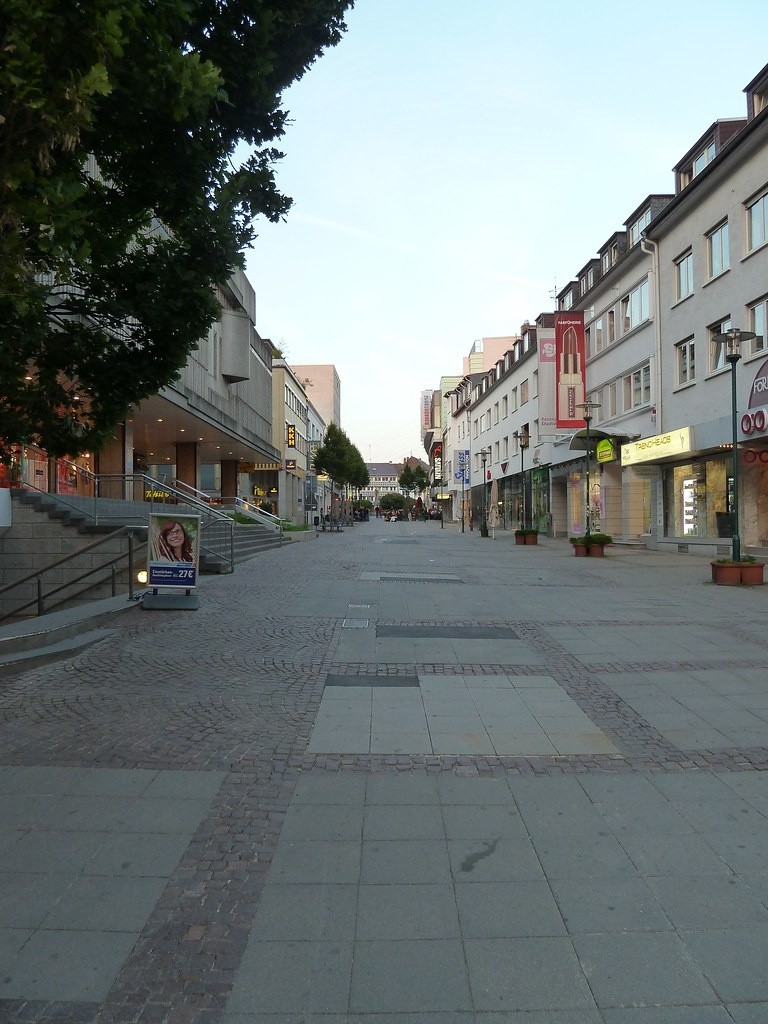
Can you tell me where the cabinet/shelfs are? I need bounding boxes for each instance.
[682,477,735,536]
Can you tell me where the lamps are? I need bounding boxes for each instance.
[301,376,314,388]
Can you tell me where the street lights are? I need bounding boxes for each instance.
[513,433,532,529]
[477,450,491,528]
[575,400,601,536]
[712,328,756,562]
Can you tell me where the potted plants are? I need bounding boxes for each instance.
[479,523,488,537]
[569,533,613,558]
[710,555,765,586]
[515,530,538,545]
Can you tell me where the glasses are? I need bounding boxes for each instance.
[166,529,185,535]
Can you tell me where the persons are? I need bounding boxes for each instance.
[375,505,383,518]
[66,459,90,497]
[158,521,194,563]
[259,501,273,515]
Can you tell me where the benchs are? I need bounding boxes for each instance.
[320,524,342,532]
